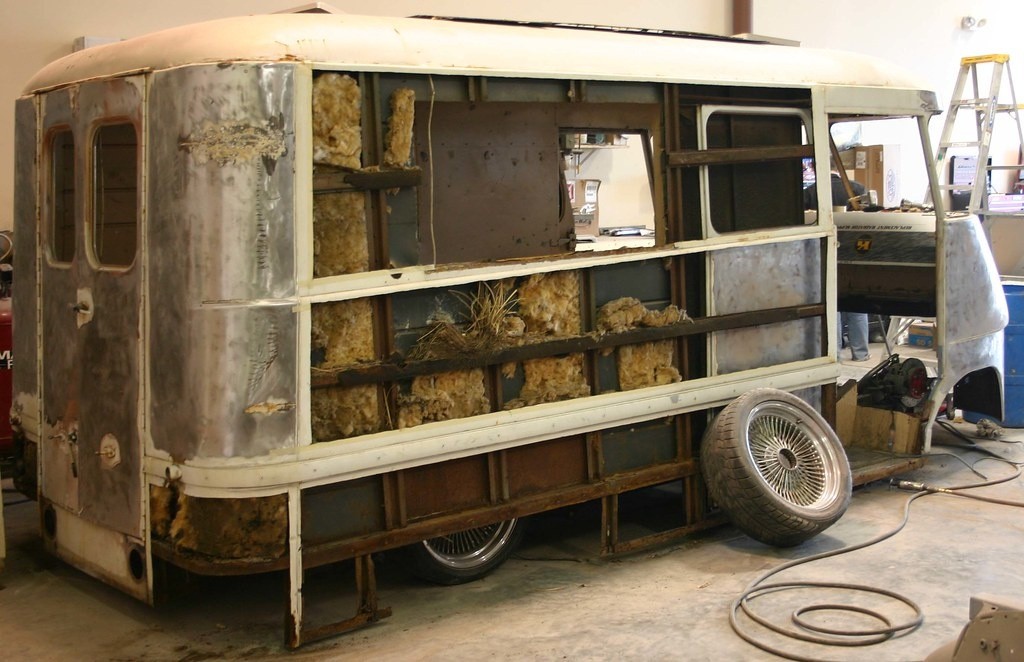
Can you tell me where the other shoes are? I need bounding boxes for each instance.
[853,353,872,362]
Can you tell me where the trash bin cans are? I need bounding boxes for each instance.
[961,280,1024,428]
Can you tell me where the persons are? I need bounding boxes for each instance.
[803,154,875,362]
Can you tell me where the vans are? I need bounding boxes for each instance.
[6,3,954,651]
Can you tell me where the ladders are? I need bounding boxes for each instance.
[922,52,1024,287]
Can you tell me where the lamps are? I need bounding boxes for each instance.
[962,16,985,32]
